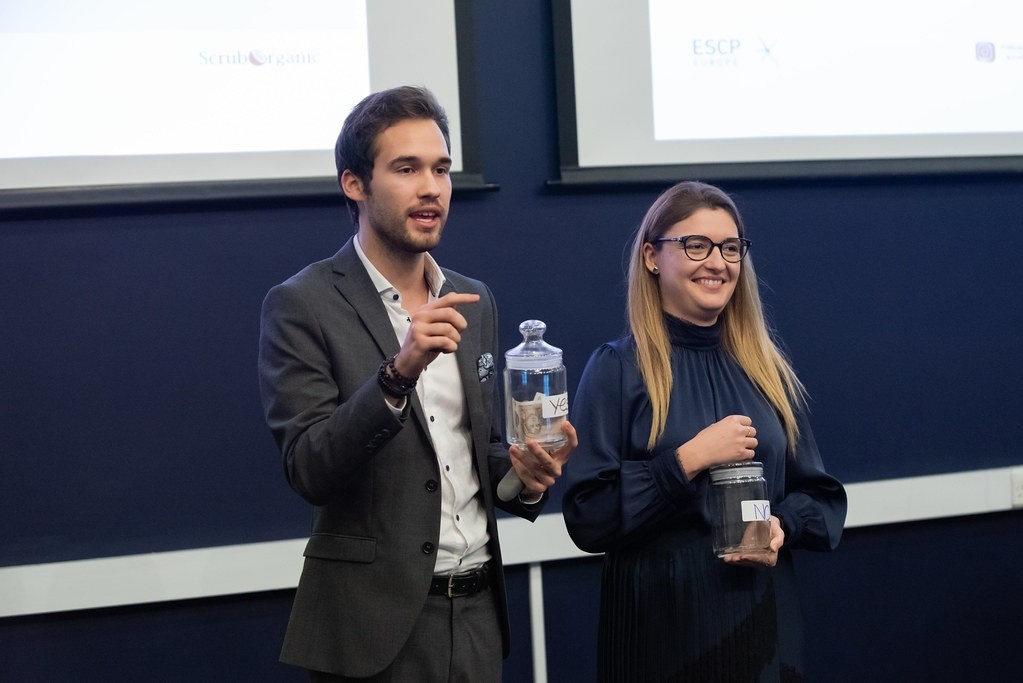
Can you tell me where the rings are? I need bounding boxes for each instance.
[745,425,752,438]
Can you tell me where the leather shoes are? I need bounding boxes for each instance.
[432,563,495,598]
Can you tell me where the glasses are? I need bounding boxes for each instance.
[656,236,753,263]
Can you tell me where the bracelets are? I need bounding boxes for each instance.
[377,354,419,398]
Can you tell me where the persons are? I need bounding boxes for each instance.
[257,87,580,682]
[562,183,849,683]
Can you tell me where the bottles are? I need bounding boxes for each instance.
[710,456,773,560]
[503,320,569,451]
